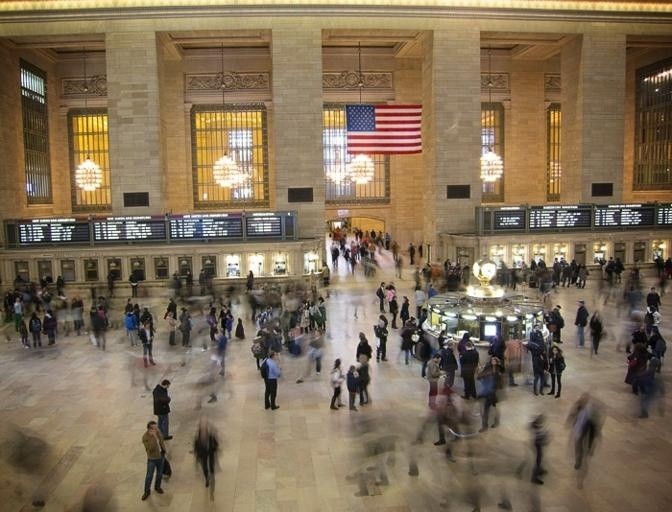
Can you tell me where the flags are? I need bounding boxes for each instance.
[345,104,423,156]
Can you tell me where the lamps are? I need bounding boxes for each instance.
[482,41,506,185]
[68,47,102,194]
[345,41,376,185]
[206,39,241,188]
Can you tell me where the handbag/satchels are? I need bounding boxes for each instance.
[261,360,269,377]
[163,460,171,475]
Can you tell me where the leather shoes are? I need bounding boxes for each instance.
[155,487,163,493]
[142,493,150,500]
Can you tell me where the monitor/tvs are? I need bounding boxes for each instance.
[482,324,499,338]
